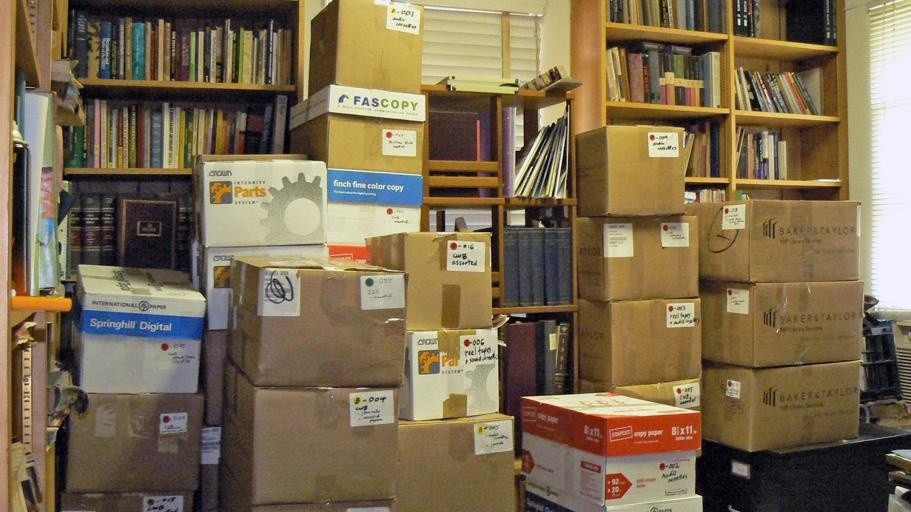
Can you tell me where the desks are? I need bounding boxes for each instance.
[692,421,909,512]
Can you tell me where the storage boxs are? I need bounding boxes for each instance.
[697,198,861,285]
[199,158,327,249]
[198,245,330,332]
[389,414,518,512]
[70,262,205,395]
[362,230,490,329]
[578,298,703,387]
[699,360,862,454]
[575,379,701,412]
[401,326,500,421]
[579,215,701,304]
[701,282,866,369]
[218,357,398,506]
[520,391,702,511]
[64,391,206,495]
[224,255,407,389]
[329,166,422,262]
[571,126,687,218]
[288,85,427,173]
[61,493,193,512]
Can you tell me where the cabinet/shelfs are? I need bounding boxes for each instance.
[53,0,307,271]
[567,0,850,199]
[419,81,582,512]
[0,1,62,512]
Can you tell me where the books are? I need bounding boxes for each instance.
[500,319,571,419]
[683,118,721,177]
[67,192,195,276]
[14,63,61,297]
[8,309,48,512]
[515,114,570,197]
[63,95,289,168]
[499,214,574,307]
[606,0,728,33]
[736,127,788,181]
[732,0,837,46]
[608,40,721,109]
[68,8,295,85]
[734,65,819,115]
[684,186,725,203]
